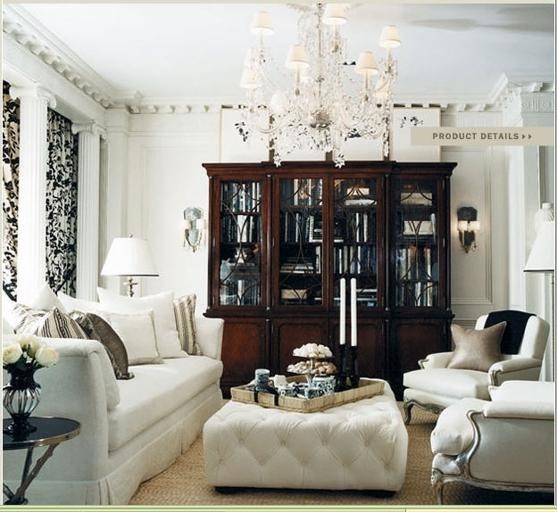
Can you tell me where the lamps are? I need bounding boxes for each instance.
[180,205,206,255]
[457,205,481,253]
[228,2,406,167]
[523,220,556,310]
[100,233,160,298]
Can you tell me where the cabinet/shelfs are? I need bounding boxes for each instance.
[330,316,456,403]
[330,160,459,318]
[201,160,330,318]
[220,315,329,401]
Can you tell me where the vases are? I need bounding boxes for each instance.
[2,381,44,438]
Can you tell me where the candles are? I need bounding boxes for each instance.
[350,277,357,346]
[340,277,347,346]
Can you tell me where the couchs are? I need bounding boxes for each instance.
[3,314,226,506]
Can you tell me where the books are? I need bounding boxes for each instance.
[392,180,438,308]
[219,179,261,306]
[278,178,322,305]
[333,179,378,307]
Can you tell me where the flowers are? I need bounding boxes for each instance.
[2,331,62,391]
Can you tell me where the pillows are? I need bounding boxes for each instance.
[3,275,206,381]
[445,321,508,373]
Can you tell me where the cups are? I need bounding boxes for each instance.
[253,368,335,399]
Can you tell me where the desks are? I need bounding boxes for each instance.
[2,415,83,507]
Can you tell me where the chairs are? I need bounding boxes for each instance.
[428,377,555,505]
[402,309,551,426]
